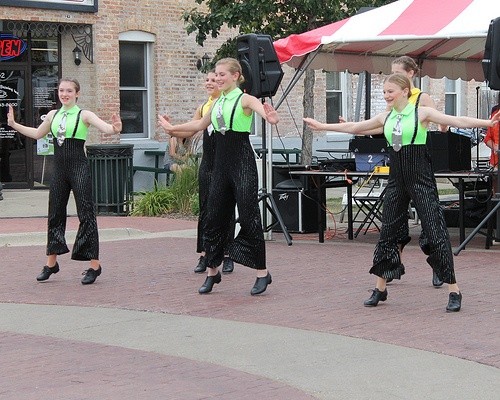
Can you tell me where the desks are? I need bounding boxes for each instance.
[315,149,354,181]
[144,150,203,193]
[290,171,486,250]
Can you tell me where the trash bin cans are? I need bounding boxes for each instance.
[85,144,135,216]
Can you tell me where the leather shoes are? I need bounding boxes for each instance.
[194,256,206,272]
[365,288,388,308]
[198,270,221,294]
[250,271,273,295]
[82,265,101,284]
[36,261,59,280]
[446,291,463,312]
[432,271,443,286]
[222,257,233,273]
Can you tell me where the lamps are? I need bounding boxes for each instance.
[72,44,82,66]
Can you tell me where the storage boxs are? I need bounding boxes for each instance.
[374,166,389,173]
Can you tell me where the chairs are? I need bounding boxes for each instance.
[350,176,392,237]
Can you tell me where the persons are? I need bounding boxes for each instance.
[158,58,280,295]
[8,78,123,284]
[301,56,500,313]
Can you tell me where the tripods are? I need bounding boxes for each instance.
[235,99,292,245]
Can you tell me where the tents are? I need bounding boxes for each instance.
[273,0,500,139]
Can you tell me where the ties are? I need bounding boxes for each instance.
[216,98,227,136]
[57,112,67,146]
[392,114,403,151]
[207,123,213,135]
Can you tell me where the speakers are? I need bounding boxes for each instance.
[482,16,500,90]
[428,130,472,173]
[237,34,284,99]
[271,162,326,233]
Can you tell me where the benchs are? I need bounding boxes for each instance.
[134,167,176,196]
